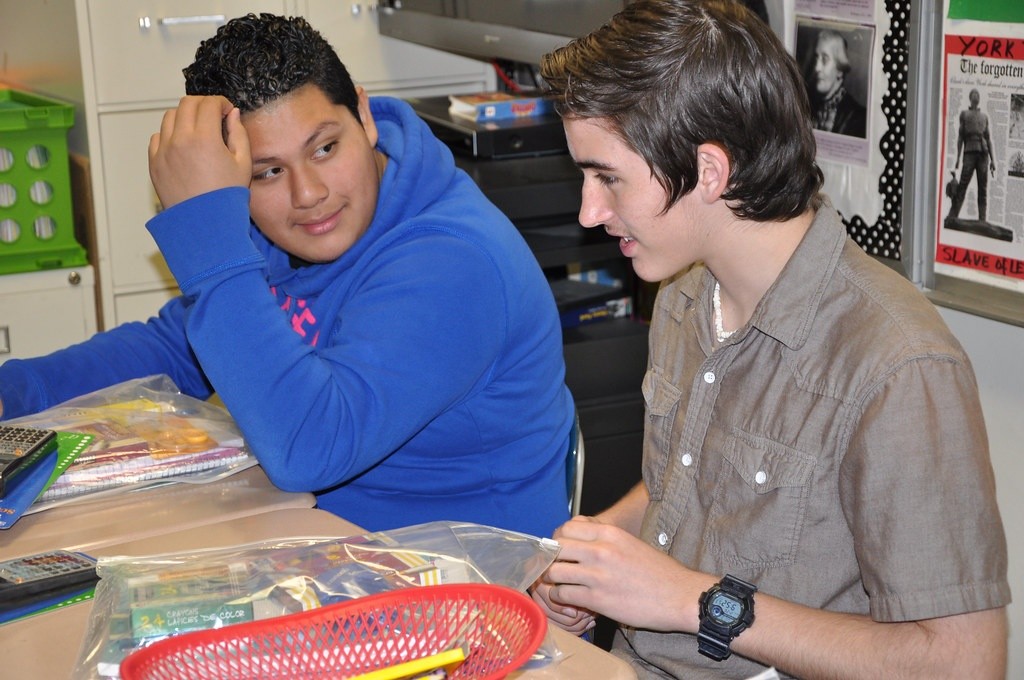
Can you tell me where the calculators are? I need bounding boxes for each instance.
[0,425,57,500]
[0,549,102,601]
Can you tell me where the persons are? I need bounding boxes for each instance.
[947,89,995,225]
[521,0,1014,680]
[0,11,577,537]
[810,25,872,141]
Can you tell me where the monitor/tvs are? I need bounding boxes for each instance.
[376,0,625,94]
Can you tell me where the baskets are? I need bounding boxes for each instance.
[0,89,90,277]
[119,582,550,680]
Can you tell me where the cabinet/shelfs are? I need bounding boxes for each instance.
[414,157,659,515]
[0,0,380,364]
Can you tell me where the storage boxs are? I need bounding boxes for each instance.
[0,88,88,275]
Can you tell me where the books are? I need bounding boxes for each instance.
[9,396,553,680]
[444,89,548,122]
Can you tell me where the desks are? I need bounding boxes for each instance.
[0,393,640,680]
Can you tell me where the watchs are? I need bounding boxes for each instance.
[697,573,757,663]
